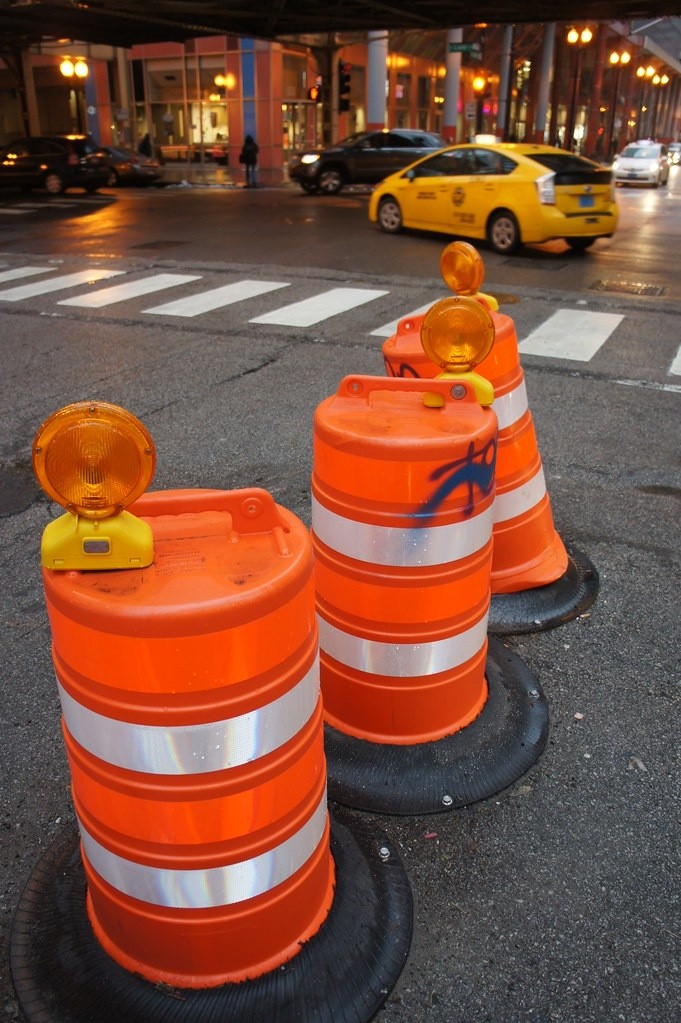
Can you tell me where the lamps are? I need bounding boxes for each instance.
[215,72,231,89]
[565,23,670,87]
[58,56,89,79]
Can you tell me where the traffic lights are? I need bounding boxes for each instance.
[338,59,350,112]
[309,84,322,101]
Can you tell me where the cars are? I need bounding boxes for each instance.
[667,142,681,165]
[613,139,669,189]
[100,145,162,187]
[368,140,618,255]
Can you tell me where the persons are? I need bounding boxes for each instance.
[140,133,152,158]
[242,135,259,188]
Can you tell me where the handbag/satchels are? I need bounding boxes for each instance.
[239,154,247,164]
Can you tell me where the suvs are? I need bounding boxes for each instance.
[291,126,444,193]
[0,131,110,195]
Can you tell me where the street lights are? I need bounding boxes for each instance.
[651,74,669,138]
[636,65,654,138]
[607,51,631,159]
[61,59,88,133]
[565,25,592,151]
